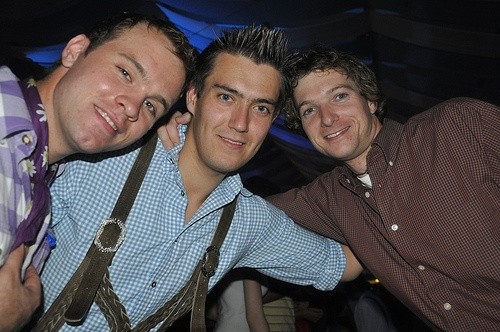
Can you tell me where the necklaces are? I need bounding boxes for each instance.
[356,169,366,176]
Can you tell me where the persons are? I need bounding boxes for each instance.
[40,23,500,332]
[0,12,199,332]
